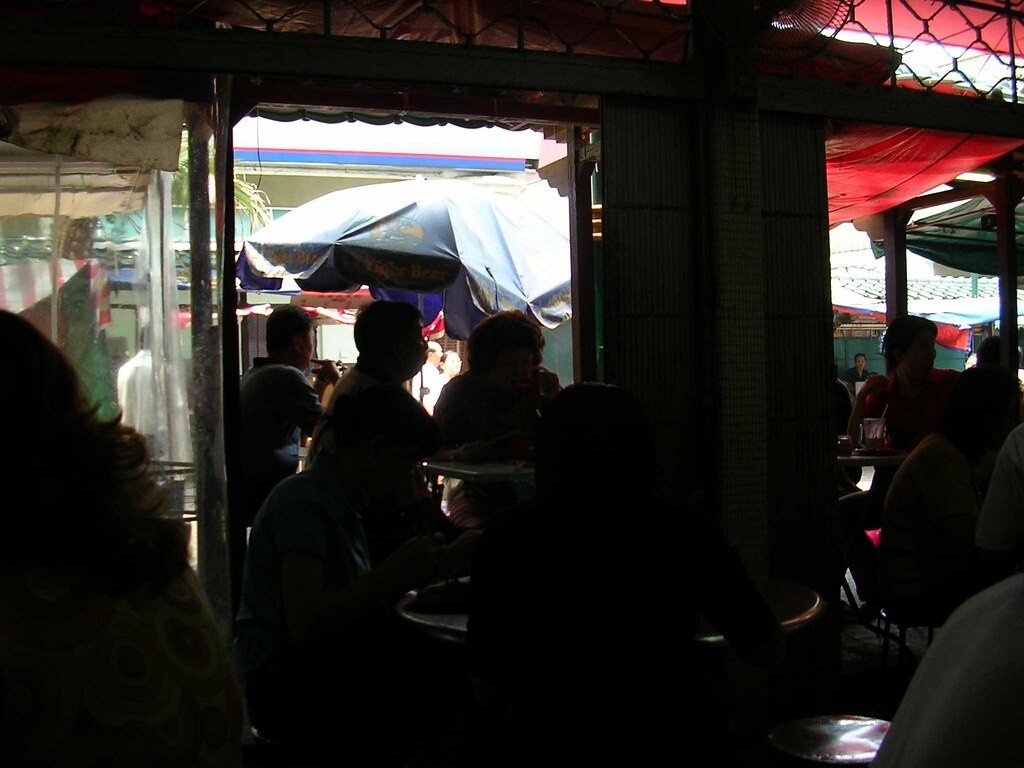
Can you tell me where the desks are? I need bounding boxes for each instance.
[415,459,536,531]
[395,565,824,768]
[836,454,906,505]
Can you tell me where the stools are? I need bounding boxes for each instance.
[760,713,891,768]
[876,608,935,687]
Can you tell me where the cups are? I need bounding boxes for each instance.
[863,416,886,448]
[837,434,851,455]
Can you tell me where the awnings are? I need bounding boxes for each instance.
[107,268,301,296]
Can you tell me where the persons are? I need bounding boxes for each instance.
[243,305,341,528]
[842,354,871,385]
[0,310,243,767]
[976,338,1021,372]
[878,366,1024,626]
[237,301,783,768]
[871,574,1024,768]
[832,365,852,421]
[117,324,191,521]
[846,318,962,450]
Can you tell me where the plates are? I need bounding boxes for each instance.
[838,456,906,467]
[858,446,899,456]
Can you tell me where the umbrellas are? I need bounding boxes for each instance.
[235,173,571,341]
